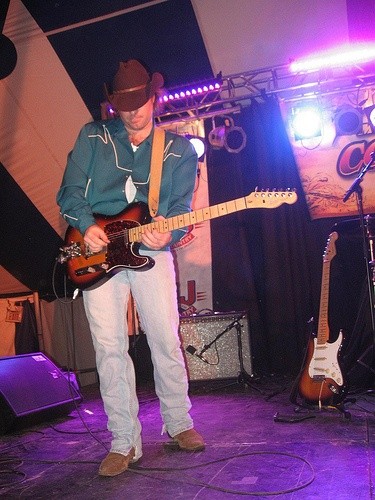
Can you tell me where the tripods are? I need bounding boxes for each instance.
[343,151,375,379]
[200,311,265,396]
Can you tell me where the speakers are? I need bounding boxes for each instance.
[178,311,253,386]
[0,352,81,434]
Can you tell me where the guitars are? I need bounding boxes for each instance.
[55,187,297,291]
[290,231,347,410]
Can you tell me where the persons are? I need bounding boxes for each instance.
[57,59,206,477]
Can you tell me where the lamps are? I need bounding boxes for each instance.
[209,114,247,154]
[330,104,363,136]
[183,125,208,163]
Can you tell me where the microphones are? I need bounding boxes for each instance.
[186,345,210,364]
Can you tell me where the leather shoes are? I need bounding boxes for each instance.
[98,447,138,477]
[167,427,204,451]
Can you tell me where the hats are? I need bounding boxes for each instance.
[104,61,164,112]
